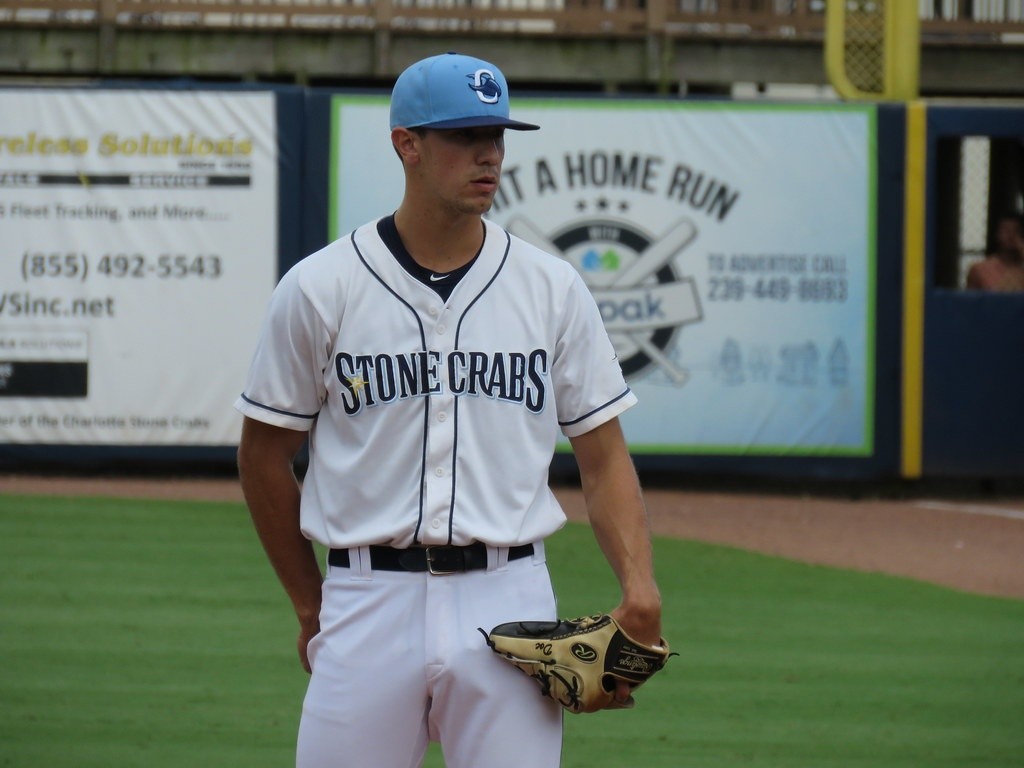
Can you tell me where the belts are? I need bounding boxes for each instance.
[328,541,534,575]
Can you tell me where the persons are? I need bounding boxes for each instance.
[968,211,1024,290]
[231,52,673,767]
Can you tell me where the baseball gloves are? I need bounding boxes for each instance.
[474,610,683,717]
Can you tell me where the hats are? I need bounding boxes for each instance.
[390,52,541,132]
